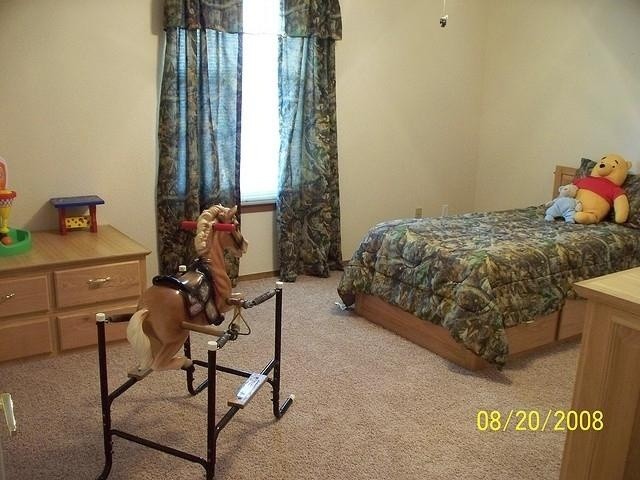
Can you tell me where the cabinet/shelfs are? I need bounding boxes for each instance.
[0,223,153,369]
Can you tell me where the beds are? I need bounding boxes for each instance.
[337,164,640,371]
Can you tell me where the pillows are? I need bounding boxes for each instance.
[571,158,640,229]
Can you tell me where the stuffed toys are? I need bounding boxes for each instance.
[545,184,583,224]
[570,154,632,224]
